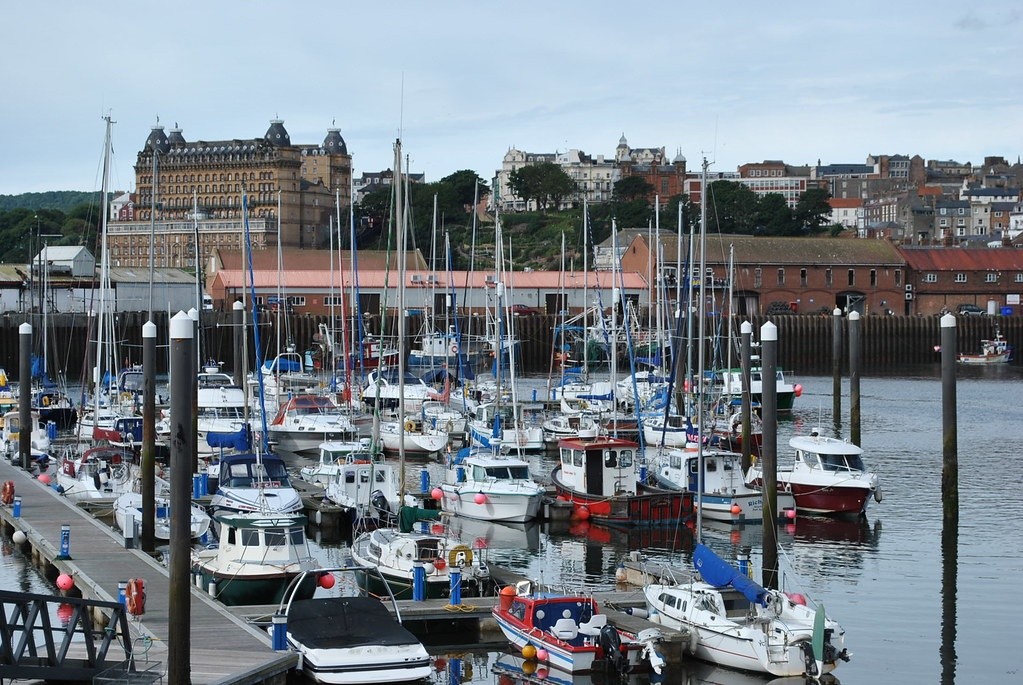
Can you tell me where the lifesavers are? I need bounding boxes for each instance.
[1,481,11,503]
[125,579,138,614]
[457,559,465,566]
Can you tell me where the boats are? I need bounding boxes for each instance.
[0,68,886,685]
[959,329,1011,364]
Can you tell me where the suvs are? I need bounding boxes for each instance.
[955,303,987,317]
